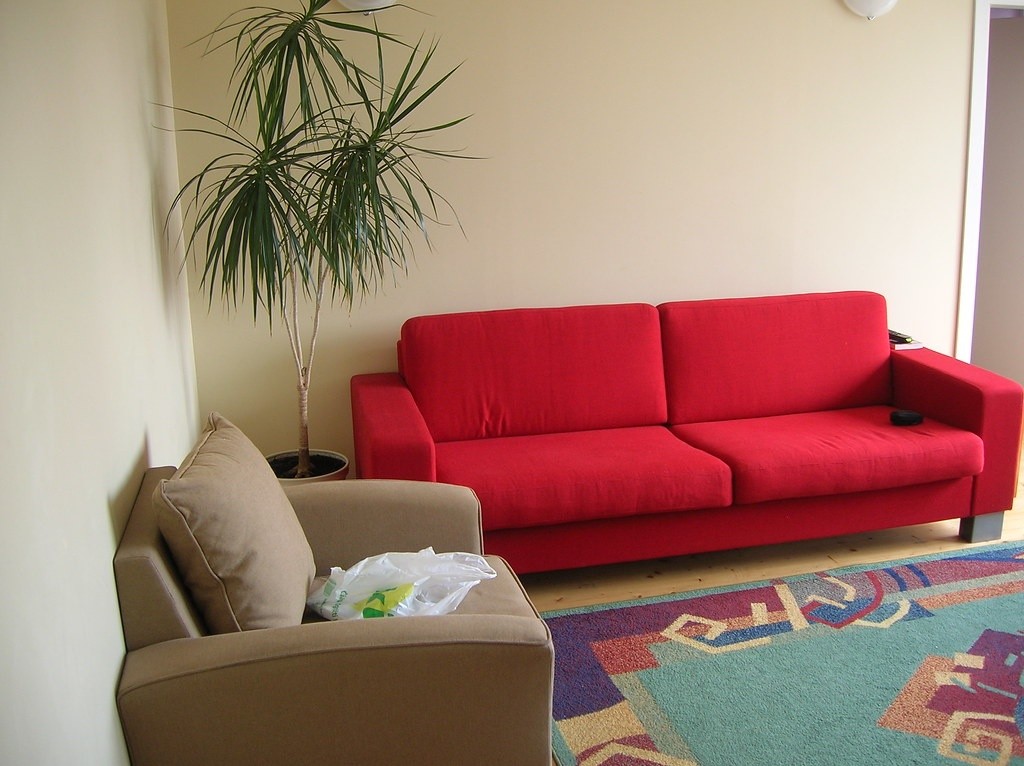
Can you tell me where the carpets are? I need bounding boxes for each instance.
[538,540,1024,766]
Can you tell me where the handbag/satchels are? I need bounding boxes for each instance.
[306,545,497,622]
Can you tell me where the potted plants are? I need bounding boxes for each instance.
[150,0,480,489]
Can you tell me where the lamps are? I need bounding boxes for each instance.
[845,0,898,22]
[336,0,397,16]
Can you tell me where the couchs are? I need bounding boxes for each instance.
[348,290,1024,597]
[108,460,557,766]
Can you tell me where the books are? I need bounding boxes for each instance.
[889,339,924,351]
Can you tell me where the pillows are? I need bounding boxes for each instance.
[154,410,319,634]
